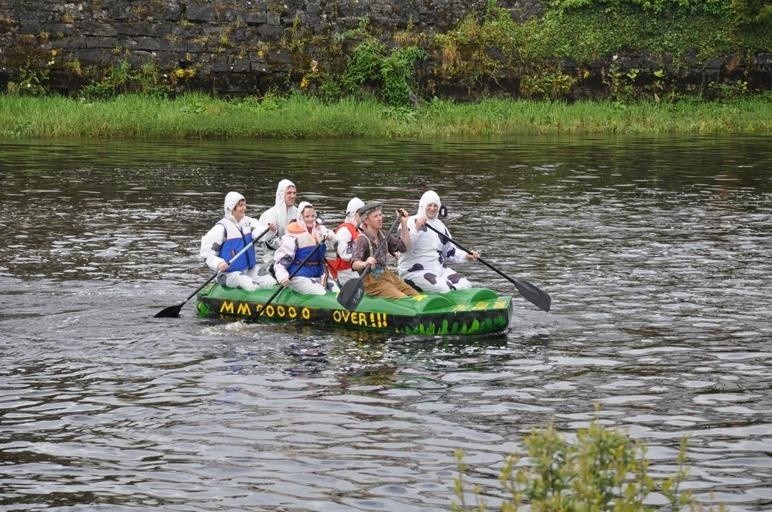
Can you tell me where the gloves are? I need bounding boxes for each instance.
[194,276,525,345]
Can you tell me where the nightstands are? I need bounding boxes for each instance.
[337,209,406,313]
[414,218,550,312]
[154,223,270,318]
[243,235,325,325]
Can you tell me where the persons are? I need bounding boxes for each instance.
[397,189,480,293]
[334,197,366,287]
[259,179,298,276]
[350,201,421,300]
[202,191,278,290]
[274,202,338,296]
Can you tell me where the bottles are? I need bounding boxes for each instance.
[356,200,383,217]
